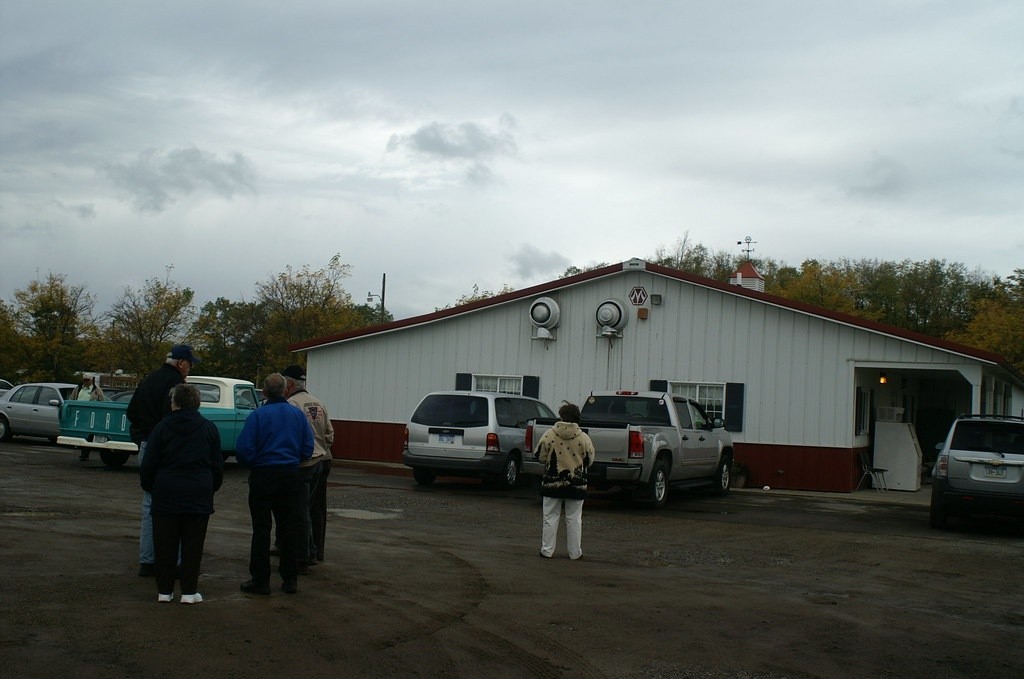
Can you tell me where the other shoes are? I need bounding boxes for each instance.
[312,560,319,565]
[176,567,181,580]
[300,567,309,575]
[280,581,298,593]
[139,563,155,576]
[158,593,174,602]
[180,593,203,604]
[270,548,280,555]
[318,555,324,561]
[240,580,270,595]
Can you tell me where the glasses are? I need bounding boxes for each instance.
[177,359,194,369]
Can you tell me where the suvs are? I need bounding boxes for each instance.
[931,414,1024,530]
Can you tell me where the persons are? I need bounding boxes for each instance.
[140,384,223,604]
[125,344,199,578]
[236,373,314,594]
[269,365,334,575]
[534,404,595,560]
[69,374,104,460]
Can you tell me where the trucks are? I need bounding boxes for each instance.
[56,376,262,468]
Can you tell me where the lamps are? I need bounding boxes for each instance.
[879,376,887,384]
[651,294,662,305]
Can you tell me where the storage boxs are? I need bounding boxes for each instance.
[876,407,905,422]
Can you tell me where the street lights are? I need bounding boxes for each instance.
[367,292,385,323]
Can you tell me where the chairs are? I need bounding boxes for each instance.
[855,451,888,493]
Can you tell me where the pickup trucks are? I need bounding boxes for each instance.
[521,389,735,511]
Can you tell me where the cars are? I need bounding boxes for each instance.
[0,379,15,397]
[100,388,135,404]
[0,383,113,443]
[199,387,267,405]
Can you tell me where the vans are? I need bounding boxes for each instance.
[402,390,558,492]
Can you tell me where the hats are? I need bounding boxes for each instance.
[280,365,306,381]
[166,345,200,363]
